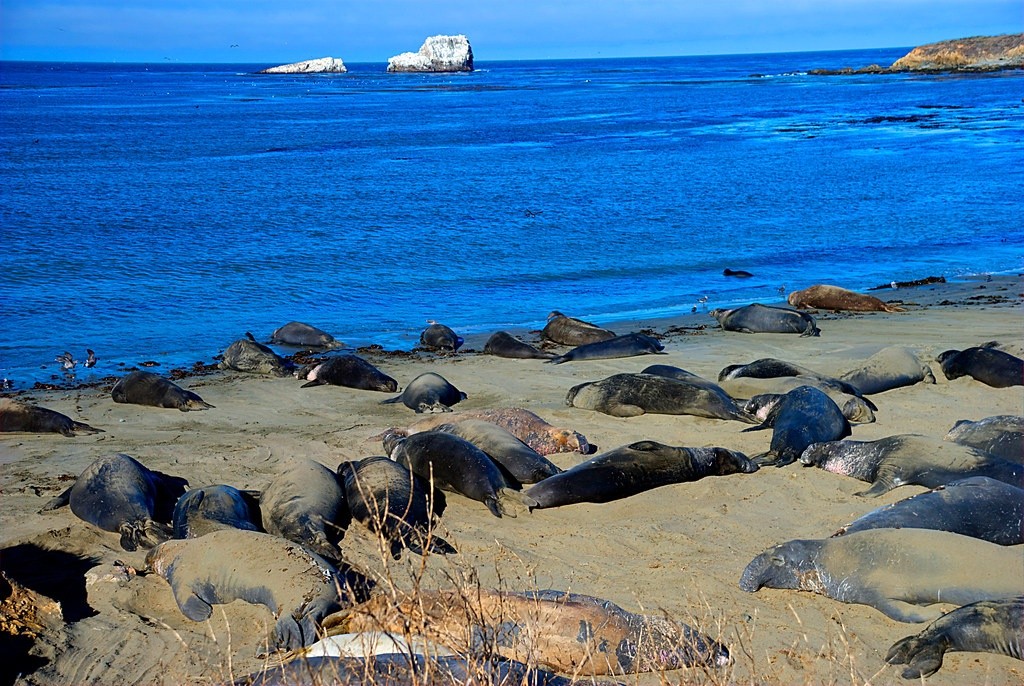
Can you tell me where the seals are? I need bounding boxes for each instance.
[0,283,1024,685]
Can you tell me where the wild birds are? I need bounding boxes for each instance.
[55,351,79,370]
[83,349,100,368]
